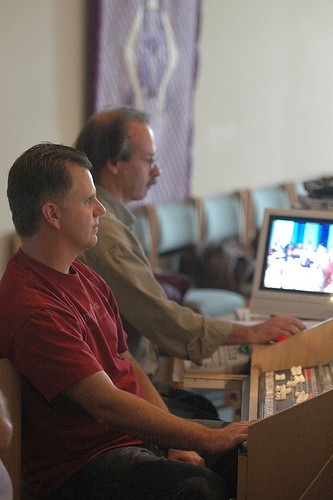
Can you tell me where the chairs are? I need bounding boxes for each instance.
[12,180,305,272]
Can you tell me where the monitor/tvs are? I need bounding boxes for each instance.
[250,208,333,320]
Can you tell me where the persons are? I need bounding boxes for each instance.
[75,107,307,485]
[0,143,261,500]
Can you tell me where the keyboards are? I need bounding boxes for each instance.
[183,315,250,374]
